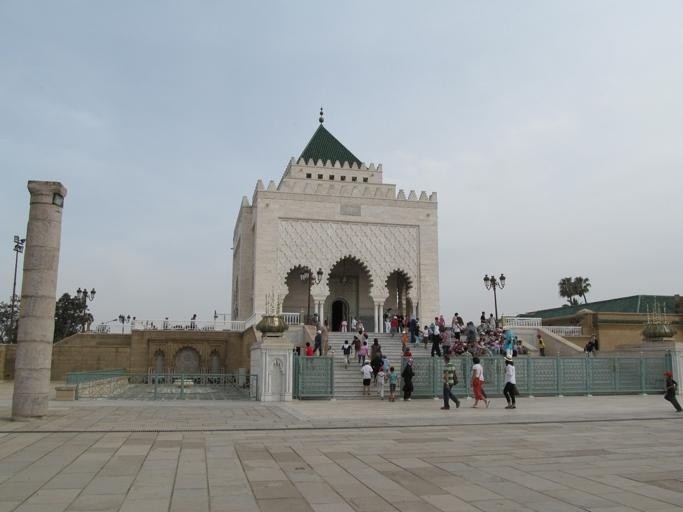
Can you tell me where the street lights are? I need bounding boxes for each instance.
[75,286,95,334]
[482,273,505,328]
[298,265,323,326]
[8,234,27,345]
[117,313,131,336]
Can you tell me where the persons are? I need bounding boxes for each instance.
[163,317,171,328]
[324,319,329,331]
[313,330,322,356]
[340,307,525,408]
[537,334,546,357]
[663,371,683,411]
[190,314,196,330]
[584,341,592,357]
[592,335,600,353]
[305,342,314,356]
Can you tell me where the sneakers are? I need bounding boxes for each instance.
[440,399,520,411]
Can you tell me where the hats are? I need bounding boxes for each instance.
[662,370,673,379]
[503,354,515,363]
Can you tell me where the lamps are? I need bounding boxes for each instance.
[340,261,349,286]
